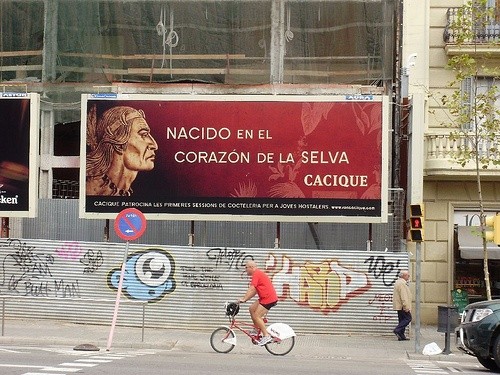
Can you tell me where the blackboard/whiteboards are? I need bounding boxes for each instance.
[450,287,470,313]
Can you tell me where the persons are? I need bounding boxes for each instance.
[237,261,280,346]
[392,270,413,340]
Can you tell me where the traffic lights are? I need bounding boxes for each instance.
[409,203,424,242]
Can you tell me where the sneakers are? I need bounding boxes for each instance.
[257,333,272,345]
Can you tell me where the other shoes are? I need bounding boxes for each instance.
[393,330,401,340]
[398,337,410,340]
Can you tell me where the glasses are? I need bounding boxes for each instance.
[406,275,409,277]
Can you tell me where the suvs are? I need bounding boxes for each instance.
[454,299,500,371]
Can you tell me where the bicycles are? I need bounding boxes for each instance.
[210,300,296,356]
